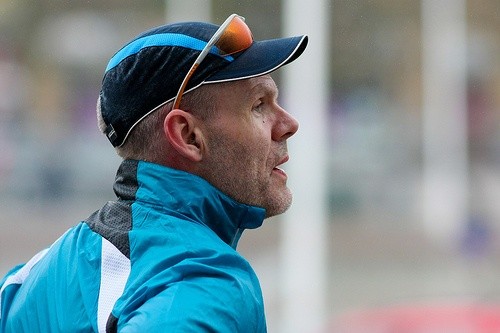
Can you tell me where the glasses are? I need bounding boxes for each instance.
[171,13,254,109]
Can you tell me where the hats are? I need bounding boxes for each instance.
[96,21,308,149]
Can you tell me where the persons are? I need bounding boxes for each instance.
[0,12,309,333]
